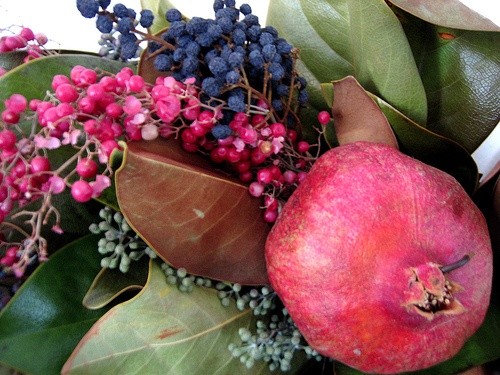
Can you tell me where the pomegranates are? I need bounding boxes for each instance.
[264,140,494,375]
